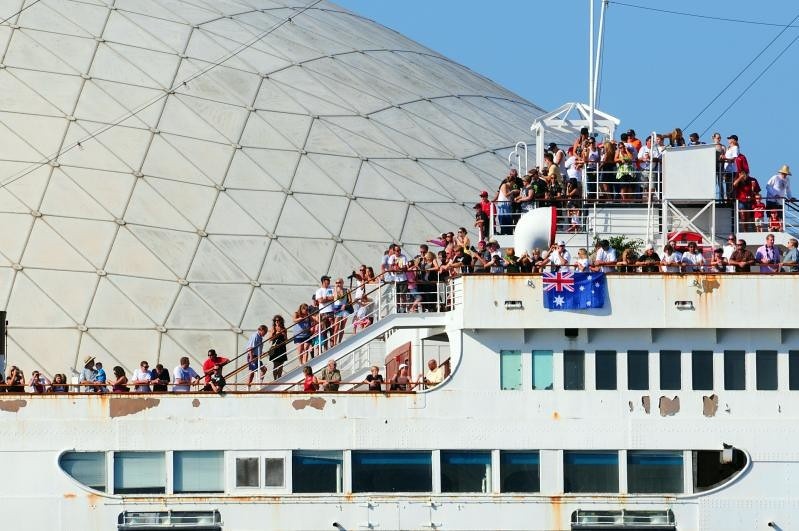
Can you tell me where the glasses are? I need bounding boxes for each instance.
[394,249,401,252]
[446,236,451,238]
[458,231,464,233]
[335,282,341,284]
[589,142,595,144]
[142,366,149,368]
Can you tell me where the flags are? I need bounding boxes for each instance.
[543,271,606,310]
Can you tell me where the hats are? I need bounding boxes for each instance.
[627,130,635,136]
[558,241,565,246]
[548,143,556,150]
[726,135,738,141]
[473,204,481,209]
[83,356,95,368]
[778,165,791,175]
[485,240,500,249]
[321,276,331,281]
[480,191,488,196]
[399,364,409,371]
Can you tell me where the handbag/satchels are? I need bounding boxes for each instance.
[345,289,354,314]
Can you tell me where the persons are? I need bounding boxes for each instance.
[246,325,269,392]
[303,366,319,391]
[380,227,470,313]
[390,364,411,391]
[364,366,383,391]
[322,360,341,391]
[425,359,444,389]
[268,315,288,380]
[0,350,229,393]
[294,265,379,367]
[471,127,799,273]
